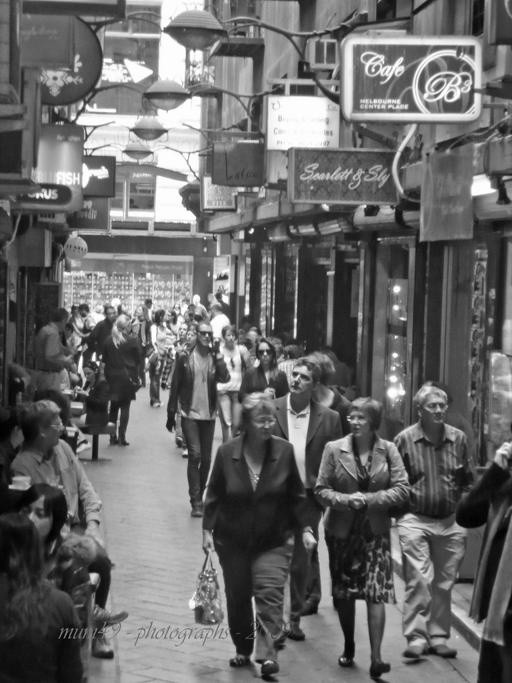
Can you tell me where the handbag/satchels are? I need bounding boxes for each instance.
[187,548,225,626]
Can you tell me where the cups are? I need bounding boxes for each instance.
[12,476,31,489]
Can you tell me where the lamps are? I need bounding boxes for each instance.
[119,8,414,186]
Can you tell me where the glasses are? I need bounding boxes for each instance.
[196,330,213,337]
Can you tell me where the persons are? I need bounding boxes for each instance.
[449,439,511,683]
[388,378,478,660]
[313,394,411,679]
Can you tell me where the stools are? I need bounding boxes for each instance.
[74,569,101,662]
[78,418,117,462]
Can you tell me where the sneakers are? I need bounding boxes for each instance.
[368,661,392,679]
[402,640,429,657]
[189,499,205,518]
[336,640,358,669]
[92,627,116,662]
[92,604,131,626]
[259,659,282,676]
[285,626,306,642]
[429,638,457,660]
[228,652,250,669]
[76,382,190,459]
